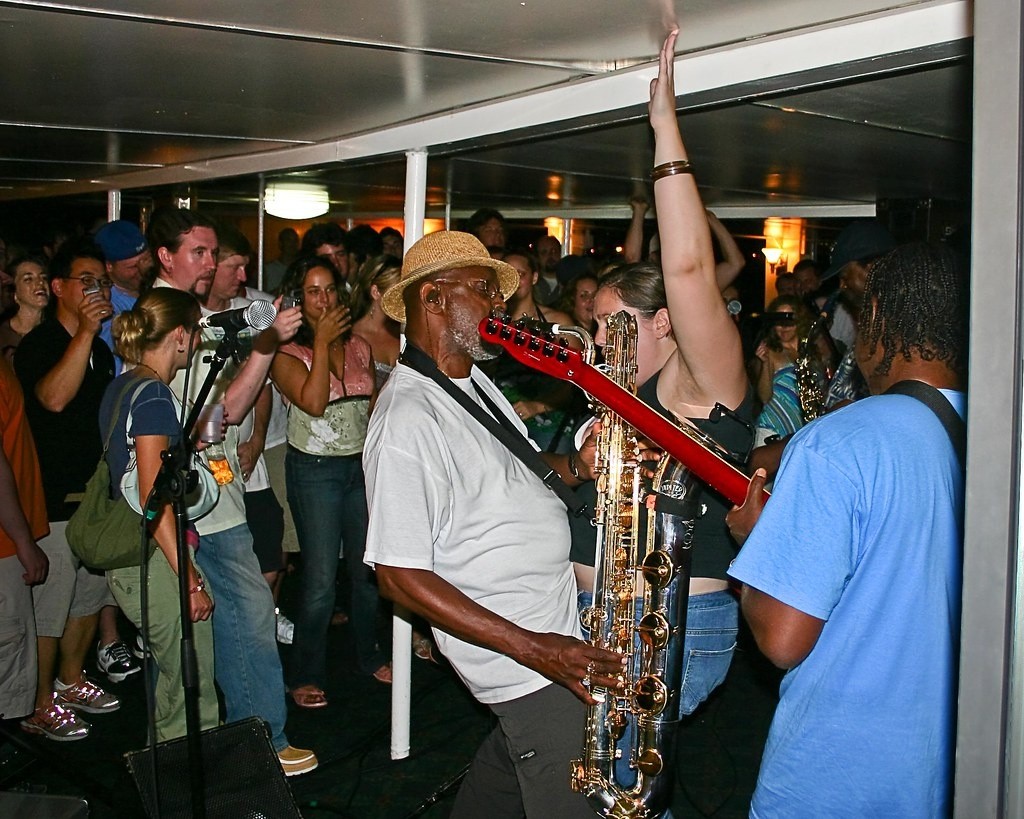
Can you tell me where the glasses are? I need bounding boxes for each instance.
[435,276,505,299]
[57,275,113,289]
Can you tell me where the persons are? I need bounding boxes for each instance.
[474,198,651,451]
[0,216,403,742]
[724,242,968,819]
[567,28,771,793]
[135,212,319,778]
[743,237,970,448]
[360,230,674,819]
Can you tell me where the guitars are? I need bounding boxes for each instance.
[476,316,774,518]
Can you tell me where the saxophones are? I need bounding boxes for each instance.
[490,309,746,818]
[792,283,846,424]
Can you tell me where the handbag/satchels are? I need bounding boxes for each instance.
[117,382,220,522]
[64,376,175,570]
[668,674,781,818]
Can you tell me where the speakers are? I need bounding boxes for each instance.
[0,728,88,819]
[114,717,303,819]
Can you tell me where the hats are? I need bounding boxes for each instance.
[94,219,147,263]
[379,231,520,323]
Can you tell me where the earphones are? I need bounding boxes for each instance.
[426,290,440,305]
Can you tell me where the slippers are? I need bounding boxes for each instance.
[292,687,327,707]
[373,665,392,683]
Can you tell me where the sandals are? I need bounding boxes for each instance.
[412,632,431,660]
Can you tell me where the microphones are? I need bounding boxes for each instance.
[194,298,277,331]
[726,300,741,317]
[820,287,847,320]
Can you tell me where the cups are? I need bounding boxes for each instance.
[197,402,225,444]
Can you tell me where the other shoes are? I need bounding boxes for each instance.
[276,744,318,777]
[276,613,294,645]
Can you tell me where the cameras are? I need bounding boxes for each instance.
[281,297,300,312]
[81,286,108,314]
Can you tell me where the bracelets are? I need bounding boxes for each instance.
[652,160,692,182]
[570,454,586,483]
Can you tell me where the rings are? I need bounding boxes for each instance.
[587,660,596,675]
[582,674,590,685]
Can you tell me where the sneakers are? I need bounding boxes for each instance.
[97,639,141,683]
[132,635,152,659]
[20,703,92,741]
[53,670,120,713]
